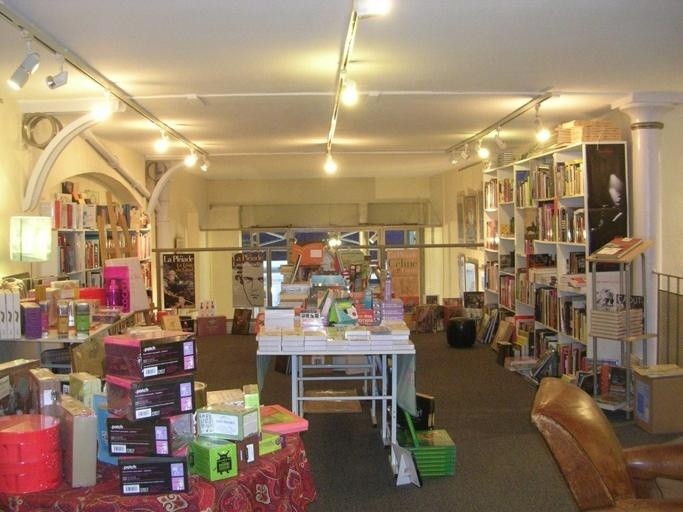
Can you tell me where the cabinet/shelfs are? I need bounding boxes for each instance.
[30,225,153,327]
[481,141,629,401]
[586,238,657,420]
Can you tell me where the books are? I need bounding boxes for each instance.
[55,194,153,326]
[634,363,683,378]
[257,318,411,352]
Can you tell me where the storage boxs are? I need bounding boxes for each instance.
[630,362,682,434]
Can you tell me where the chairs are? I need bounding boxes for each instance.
[526,376,681,512]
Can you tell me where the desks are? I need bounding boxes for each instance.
[0,429,317,512]
[256,348,416,479]
[0,308,138,374]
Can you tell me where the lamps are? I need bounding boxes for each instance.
[5,29,66,91]
[9,215,52,297]
[492,126,506,150]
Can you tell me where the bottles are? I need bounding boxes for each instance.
[107,278,119,306]
